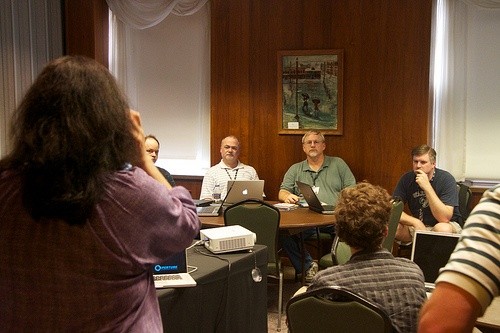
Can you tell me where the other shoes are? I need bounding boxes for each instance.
[300,262,318,285]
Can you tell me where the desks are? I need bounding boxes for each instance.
[198,201,340,289]
[155,240,272,333]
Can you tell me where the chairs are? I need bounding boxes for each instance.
[225,183,471,333]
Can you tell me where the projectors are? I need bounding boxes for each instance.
[199,225,257,254]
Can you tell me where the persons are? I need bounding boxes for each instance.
[306,181,427,333]
[142,134,176,189]
[0,55,201,333]
[392,145,464,248]
[278,129,356,285]
[417,184,500,333]
[199,135,266,203]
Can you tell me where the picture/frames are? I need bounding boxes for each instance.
[277,49,344,135]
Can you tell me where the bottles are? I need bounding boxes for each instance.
[213,184,221,204]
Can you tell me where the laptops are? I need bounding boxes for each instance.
[295,180,336,214]
[411,230,462,299]
[152,248,197,288]
[195,181,235,217]
[224,180,264,205]
